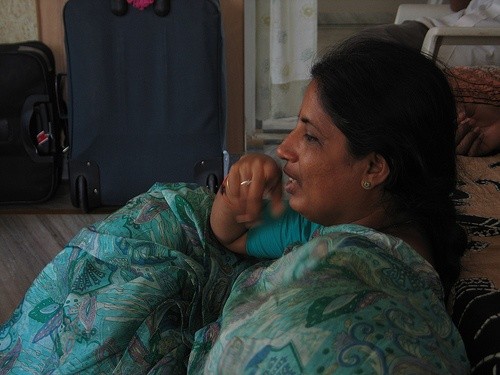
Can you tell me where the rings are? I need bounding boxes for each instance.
[240,180,251,186]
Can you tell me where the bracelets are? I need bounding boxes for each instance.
[221,175,230,204]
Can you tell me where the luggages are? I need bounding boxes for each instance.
[61,0,232,215]
[0,39,62,207]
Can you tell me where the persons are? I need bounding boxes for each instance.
[0,37,464,375]
[454,100,500,158]
